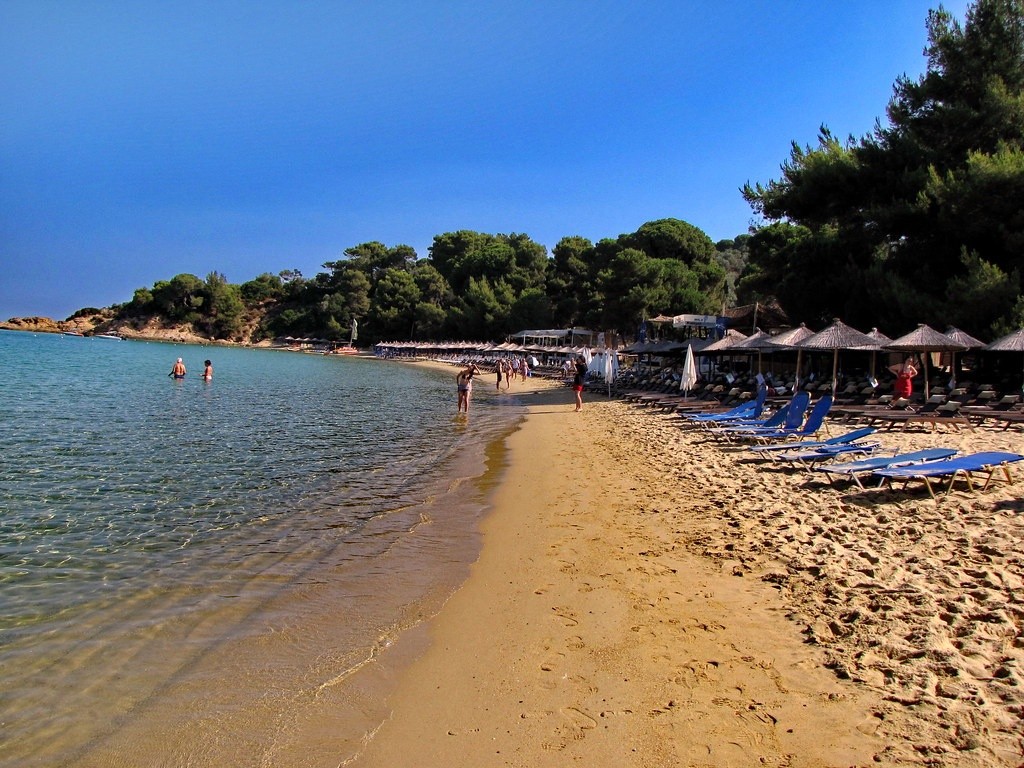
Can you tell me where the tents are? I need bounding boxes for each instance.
[509,329,585,345]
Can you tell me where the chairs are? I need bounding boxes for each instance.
[452,362,1024,501]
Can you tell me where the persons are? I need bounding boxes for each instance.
[461,354,531,390]
[199,360,213,382]
[456,363,482,412]
[570,355,588,412]
[887,355,917,407]
[173,358,187,380]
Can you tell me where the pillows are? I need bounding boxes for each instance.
[890,379,896,384]
[978,391,996,398]
[951,388,967,396]
[978,384,992,390]
[857,383,867,387]
[999,394,1019,403]
[895,397,910,407]
[930,386,945,393]
[861,387,874,393]
[786,382,794,388]
[805,383,815,390]
[944,400,962,411]
[878,395,893,402]
[845,382,856,386]
[844,385,856,393]
[911,392,922,400]
[814,381,820,385]
[927,395,946,403]
[880,383,891,389]
[818,384,830,391]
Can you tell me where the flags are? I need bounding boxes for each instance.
[351,319,358,340]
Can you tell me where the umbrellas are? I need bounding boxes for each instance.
[619,335,718,376]
[696,322,1024,403]
[374,339,621,367]
[581,346,619,401]
[679,342,696,401]
[284,336,329,343]
